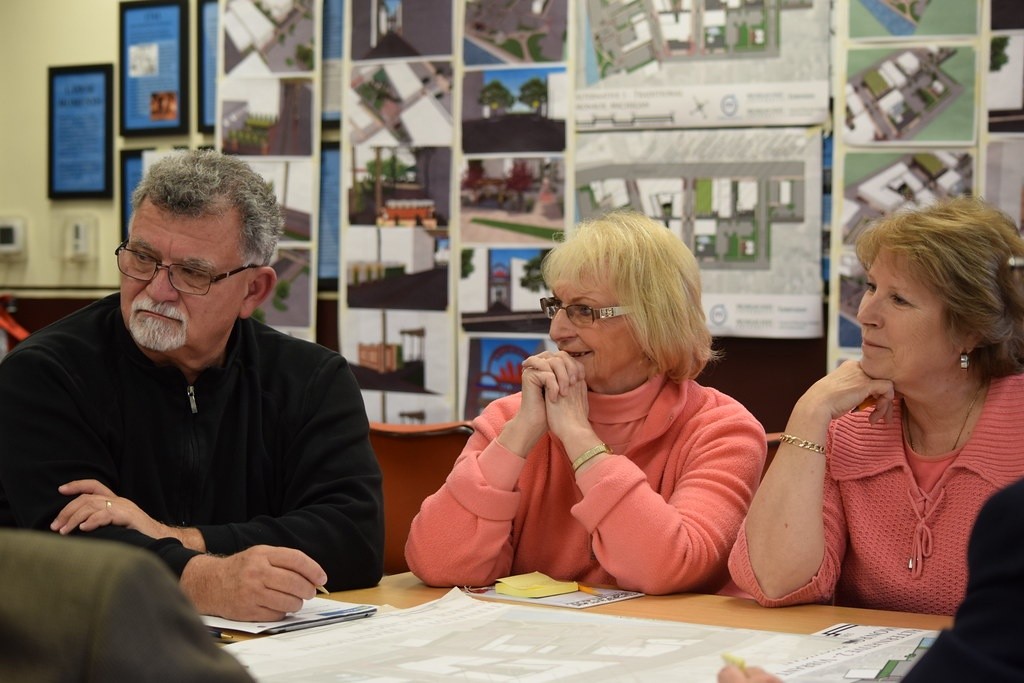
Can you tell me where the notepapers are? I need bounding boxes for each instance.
[494,570,586,599]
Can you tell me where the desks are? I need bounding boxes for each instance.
[208,572,952,683]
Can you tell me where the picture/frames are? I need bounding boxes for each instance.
[119,145,188,243]
[47,64,114,201]
[118,0,187,137]
[197,0,344,135]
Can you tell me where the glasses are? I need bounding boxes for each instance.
[114,238,262,296]
[540,297,632,327]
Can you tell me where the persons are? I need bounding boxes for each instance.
[0,148,384,623]
[720,477,1024,682]
[0,529,255,683]
[404,208,768,597]
[726,197,1024,617]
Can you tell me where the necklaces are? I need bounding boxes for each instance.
[905,379,985,454]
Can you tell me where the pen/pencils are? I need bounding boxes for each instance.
[316,584,330,595]
[206,628,234,639]
[577,584,604,597]
[720,651,746,672]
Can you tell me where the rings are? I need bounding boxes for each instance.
[105,499,112,510]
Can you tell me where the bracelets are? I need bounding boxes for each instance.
[779,433,826,455]
[572,443,614,472]
